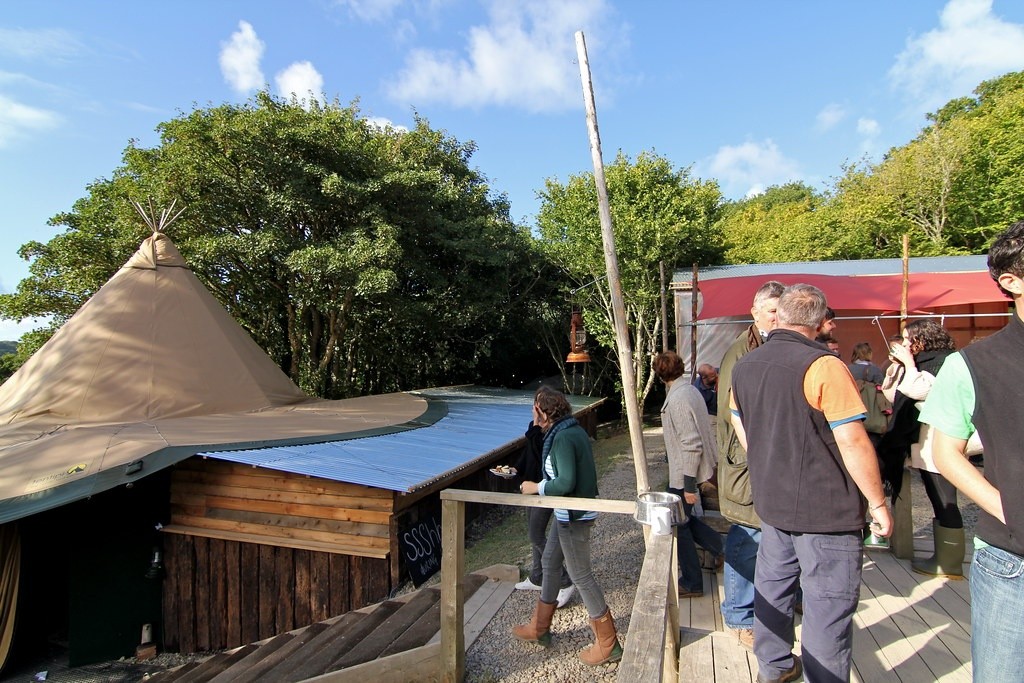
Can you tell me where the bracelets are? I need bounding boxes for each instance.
[868,497,886,510]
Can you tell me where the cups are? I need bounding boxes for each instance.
[862,522,890,550]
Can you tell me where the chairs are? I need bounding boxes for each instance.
[694,461,733,573]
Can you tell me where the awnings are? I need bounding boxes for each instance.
[690,273,1014,323]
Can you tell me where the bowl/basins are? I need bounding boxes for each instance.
[633,491,689,525]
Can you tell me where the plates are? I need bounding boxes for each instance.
[490,469,517,478]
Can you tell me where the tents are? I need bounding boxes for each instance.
[0,192,449,532]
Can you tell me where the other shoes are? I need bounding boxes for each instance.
[678,576,704,597]
[704,554,724,573]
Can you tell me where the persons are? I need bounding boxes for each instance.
[652,350,727,598]
[918,237,1024,682]
[877,319,964,580]
[510,386,576,610]
[816,307,904,485]
[727,283,895,683]
[508,387,624,666]
[694,364,718,416]
[716,280,835,654]
[677,271,857,318]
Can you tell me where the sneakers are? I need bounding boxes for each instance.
[726,624,757,654]
[514,577,543,590]
[794,599,803,615]
[756,654,802,683]
[556,584,575,608]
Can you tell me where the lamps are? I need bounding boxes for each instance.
[566,289,592,363]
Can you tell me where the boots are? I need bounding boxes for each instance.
[513,596,559,647]
[911,518,965,580]
[580,604,623,666]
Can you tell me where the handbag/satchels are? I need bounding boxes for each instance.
[855,365,892,433]
